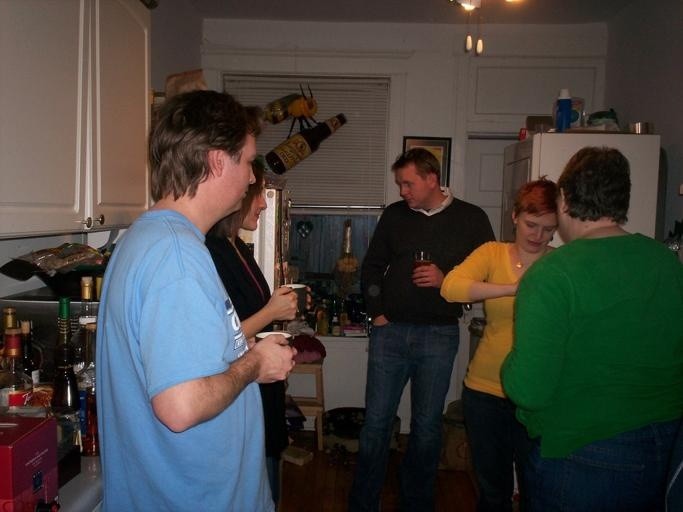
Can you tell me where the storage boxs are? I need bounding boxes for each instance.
[0,414,59,512]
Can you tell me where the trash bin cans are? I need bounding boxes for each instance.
[468,317,487,363]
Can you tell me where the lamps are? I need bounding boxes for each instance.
[453,0,487,12]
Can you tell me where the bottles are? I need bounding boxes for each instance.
[266,111,347,174]
[332,218,361,292]
[49,276,102,458]
[0,306,42,415]
[555,86,572,131]
[256,91,303,123]
[318,297,344,336]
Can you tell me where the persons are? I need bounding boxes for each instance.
[97,90,299,512]
[500,147,682,512]
[439,175,560,512]
[348,148,497,512]
[204,163,312,512]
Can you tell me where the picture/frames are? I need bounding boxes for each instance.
[401,135,452,189]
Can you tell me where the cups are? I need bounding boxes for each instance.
[412,249,433,267]
[281,282,308,318]
[255,331,293,348]
[628,120,650,134]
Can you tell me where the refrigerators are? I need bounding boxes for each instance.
[238,189,291,294]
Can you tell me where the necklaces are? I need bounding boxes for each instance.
[513,246,548,268]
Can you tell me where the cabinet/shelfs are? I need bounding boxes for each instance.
[0,0,150,247]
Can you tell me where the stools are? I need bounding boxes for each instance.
[280,333,328,452]
